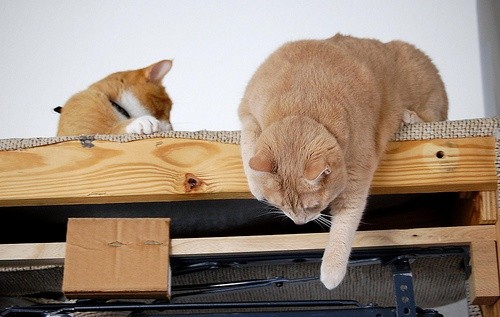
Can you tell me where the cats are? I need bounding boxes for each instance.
[56,58,173,136]
[236,33,449,291]
